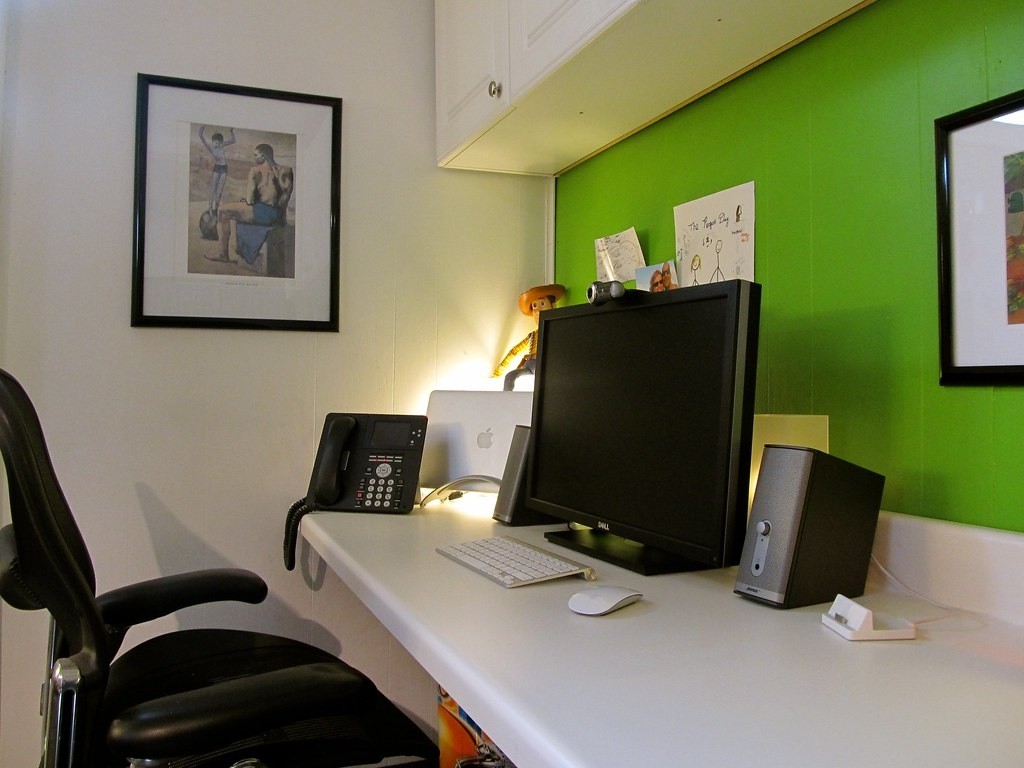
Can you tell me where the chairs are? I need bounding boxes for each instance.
[0,370,438,767]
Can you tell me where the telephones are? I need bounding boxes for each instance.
[306,412,427,514]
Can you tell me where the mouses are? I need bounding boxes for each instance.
[568,586,643,616]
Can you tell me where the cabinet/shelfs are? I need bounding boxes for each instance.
[432,2,879,178]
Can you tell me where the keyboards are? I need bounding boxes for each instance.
[435,535,597,588]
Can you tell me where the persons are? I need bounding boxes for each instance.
[489,284,565,392]
[649,262,678,292]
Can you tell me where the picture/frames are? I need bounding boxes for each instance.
[131,72,345,332]
[932,87,1023,388]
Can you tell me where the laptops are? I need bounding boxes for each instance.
[418,390,533,492]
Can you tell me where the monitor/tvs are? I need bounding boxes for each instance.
[527,279,762,576]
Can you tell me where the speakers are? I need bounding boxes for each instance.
[733,444,886,609]
[491,425,570,526]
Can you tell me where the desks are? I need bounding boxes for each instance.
[295,486,1024,767]
